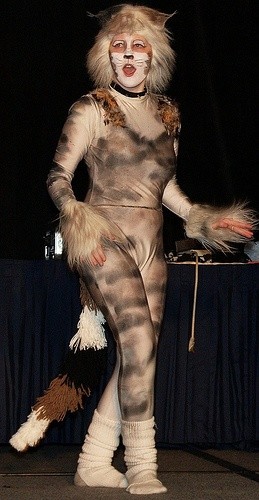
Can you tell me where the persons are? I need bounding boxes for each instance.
[10,3,253,494]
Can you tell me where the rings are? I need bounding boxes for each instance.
[232,225,234,230]
